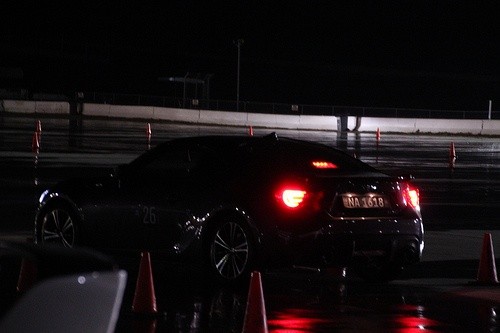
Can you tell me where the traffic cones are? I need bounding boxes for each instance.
[124,249,169,318]
[466,231,500,286]
[241,271,269,333]
[32,132,39,148]
[249,125,253,136]
[375,128,380,141]
[35,120,42,135]
[146,123,152,134]
[447,142,456,168]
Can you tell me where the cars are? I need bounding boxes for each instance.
[30,134,426,288]
